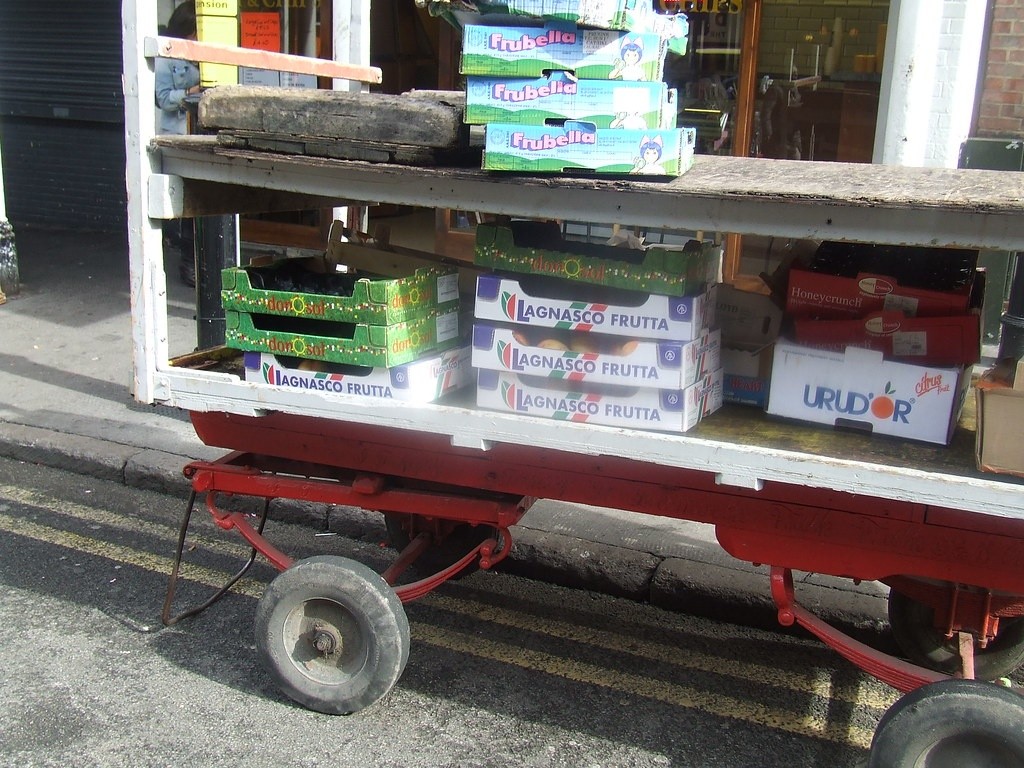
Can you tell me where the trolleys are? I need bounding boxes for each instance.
[157,405,1024,768]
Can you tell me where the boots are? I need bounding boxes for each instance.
[180,237,196,287]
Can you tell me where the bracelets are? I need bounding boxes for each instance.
[186,88,189,94]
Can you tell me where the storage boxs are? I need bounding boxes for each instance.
[785,239,980,321]
[482,123,696,177]
[458,24,668,82]
[243,342,472,404]
[793,266,988,363]
[472,323,721,390]
[225,299,462,369]
[473,220,721,299]
[221,255,460,327]
[463,76,679,129]
[474,275,719,341]
[439,0,690,58]
[476,368,723,433]
[974,367,1024,478]
[766,333,975,445]
[721,338,775,409]
[716,239,819,353]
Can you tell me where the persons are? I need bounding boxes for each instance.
[154,0,200,289]
